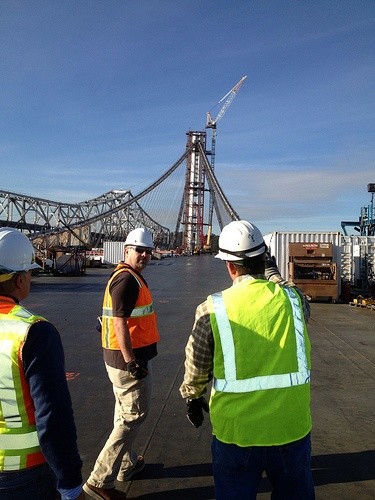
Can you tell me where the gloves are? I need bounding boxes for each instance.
[126,357,149,380]
[262,251,283,280]
[185,396,210,428]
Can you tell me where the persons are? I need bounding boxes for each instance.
[82,228,159,500]
[179,220,316,500]
[0,227,85,500]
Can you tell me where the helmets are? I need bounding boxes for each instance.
[0,227,44,272]
[123,227,154,249]
[214,220,268,262]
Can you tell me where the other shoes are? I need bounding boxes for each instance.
[117,455,145,482]
[82,482,121,500]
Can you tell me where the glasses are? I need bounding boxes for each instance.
[126,246,152,256]
